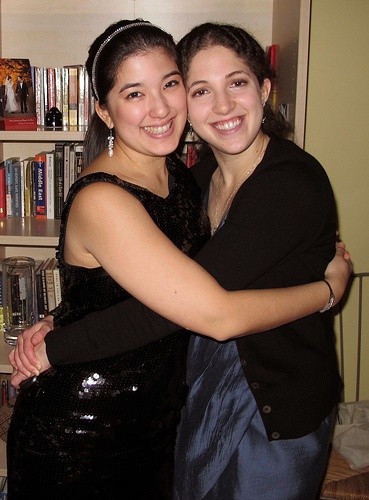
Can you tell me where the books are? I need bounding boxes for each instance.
[0,258,63,331]
[35,64,95,132]
[262,45,279,118]
[1,143,88,220]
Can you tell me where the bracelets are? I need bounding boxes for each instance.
[320,279,333,316]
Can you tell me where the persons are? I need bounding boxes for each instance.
[8,17,354,500]
[5,74,28,113]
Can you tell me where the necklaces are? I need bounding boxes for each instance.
[213,136,266,234]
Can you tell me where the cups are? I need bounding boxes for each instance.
[2,256,38,345]
